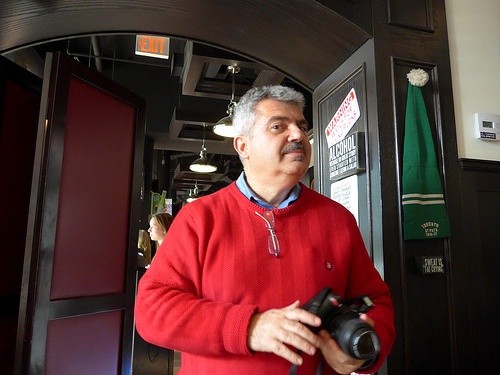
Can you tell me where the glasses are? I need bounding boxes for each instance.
[255,211,280,256]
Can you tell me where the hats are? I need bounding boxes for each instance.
[402,68,452,241]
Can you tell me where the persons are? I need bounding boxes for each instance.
[134,85,396,375]
[148,212,173,245]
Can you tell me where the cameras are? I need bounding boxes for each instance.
[300,288,380,360]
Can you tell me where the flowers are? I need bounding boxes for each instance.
[406,68,430,87]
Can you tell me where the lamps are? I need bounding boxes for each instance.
[188,122,219,173]
[212,63,241,138]
